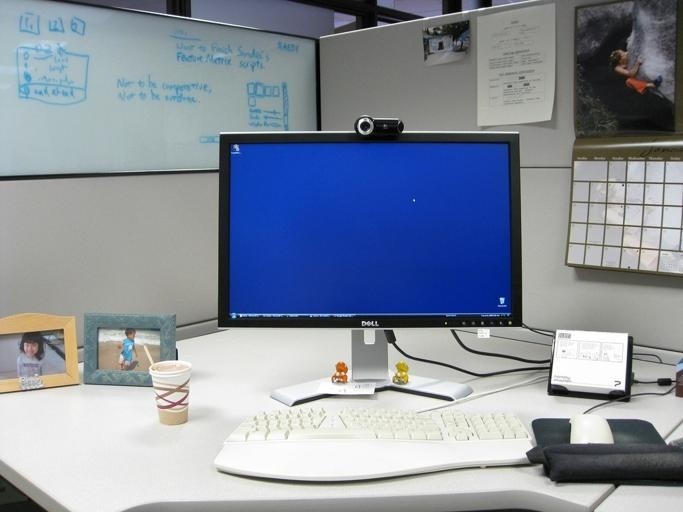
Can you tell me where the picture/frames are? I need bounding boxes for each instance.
[0,313,81,396]
[83,312,177,388]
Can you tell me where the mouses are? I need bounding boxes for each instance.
[569,415,614,446]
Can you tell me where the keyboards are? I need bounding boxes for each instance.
[214,409,537,482]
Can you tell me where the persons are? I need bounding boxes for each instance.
[610,48,663,94]
[117,327,139,371]
[17,331,45,375]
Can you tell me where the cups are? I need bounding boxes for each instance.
[148,360,193,428]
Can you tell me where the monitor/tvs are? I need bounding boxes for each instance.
[217,131,523,408]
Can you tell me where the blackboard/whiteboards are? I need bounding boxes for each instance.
[0,0,321,181]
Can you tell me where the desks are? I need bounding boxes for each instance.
[2,327,682,510]
